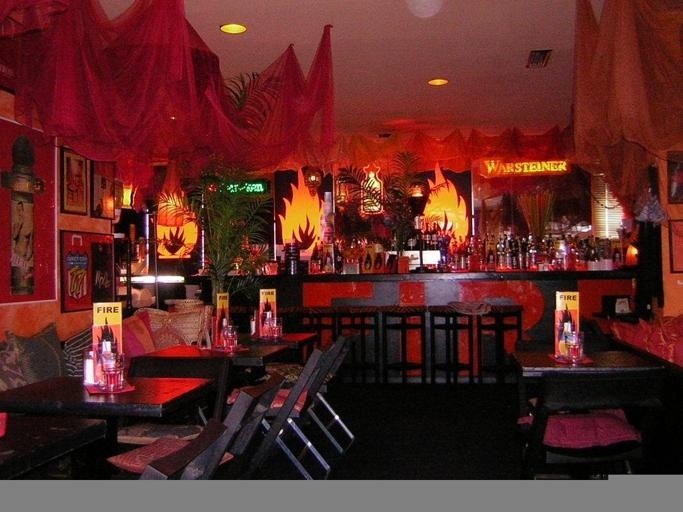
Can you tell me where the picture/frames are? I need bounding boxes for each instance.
[668,219,683,273]
[666,150,682,204]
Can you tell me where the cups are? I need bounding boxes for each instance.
[99,352,125,392]
[562,330,585,368]
[221,325,240,355]
[269,317,283,338]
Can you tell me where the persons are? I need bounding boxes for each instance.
[11,200,35,299]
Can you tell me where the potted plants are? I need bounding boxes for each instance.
[175,158,277,354]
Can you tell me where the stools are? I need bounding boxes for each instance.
[229,301,524,399]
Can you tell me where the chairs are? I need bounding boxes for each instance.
[105,327,363,480]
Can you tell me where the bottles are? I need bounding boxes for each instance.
[309,223,446,275]
[447,234,619,272]
[284,242,300,276]
[249,310,256,335]
[84,350,93,384]
[362,163,381,213]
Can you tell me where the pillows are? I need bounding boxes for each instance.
[0,304,214,392]
[517,408,642,449]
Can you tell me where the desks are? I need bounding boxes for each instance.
[0,333,317,480]
[508,347,683,479]
